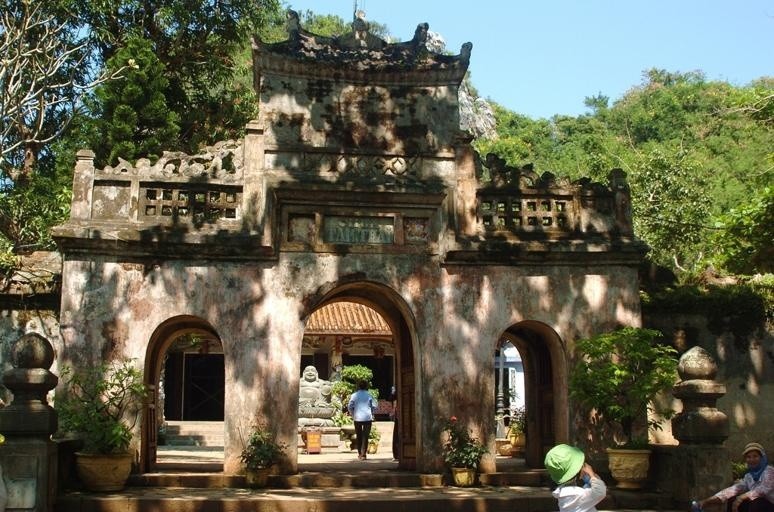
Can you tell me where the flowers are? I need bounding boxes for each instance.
[441,416,486,469]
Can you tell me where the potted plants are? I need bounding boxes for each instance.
[560,326,679,491]
[240,424,288,487]
[45,359,155,491]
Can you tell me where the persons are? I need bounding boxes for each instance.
[690,442,774,512]
[348,379,380,461]
[297,365,346,419]
[543,442,608,512]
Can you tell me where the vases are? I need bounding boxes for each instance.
[451,465,476,485]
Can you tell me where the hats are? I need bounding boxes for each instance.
[741,442,766,456]
[544,443,586,485]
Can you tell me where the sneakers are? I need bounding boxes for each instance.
[358,454,366,460]
